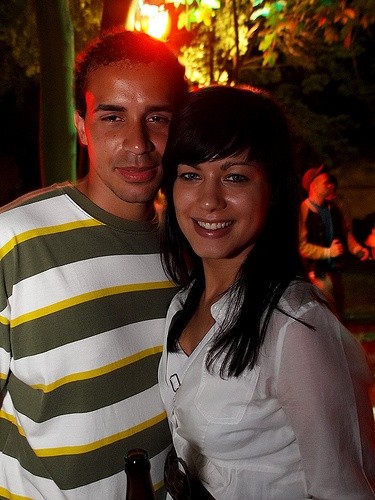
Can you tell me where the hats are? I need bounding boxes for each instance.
[300,162,326,191]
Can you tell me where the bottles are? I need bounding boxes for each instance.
[124,448,156,500]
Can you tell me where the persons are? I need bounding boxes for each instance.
[299,163,375,312]
[159,85,375,500]
[0,30,191,500]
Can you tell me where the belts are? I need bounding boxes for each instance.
[164,447,215,500]
